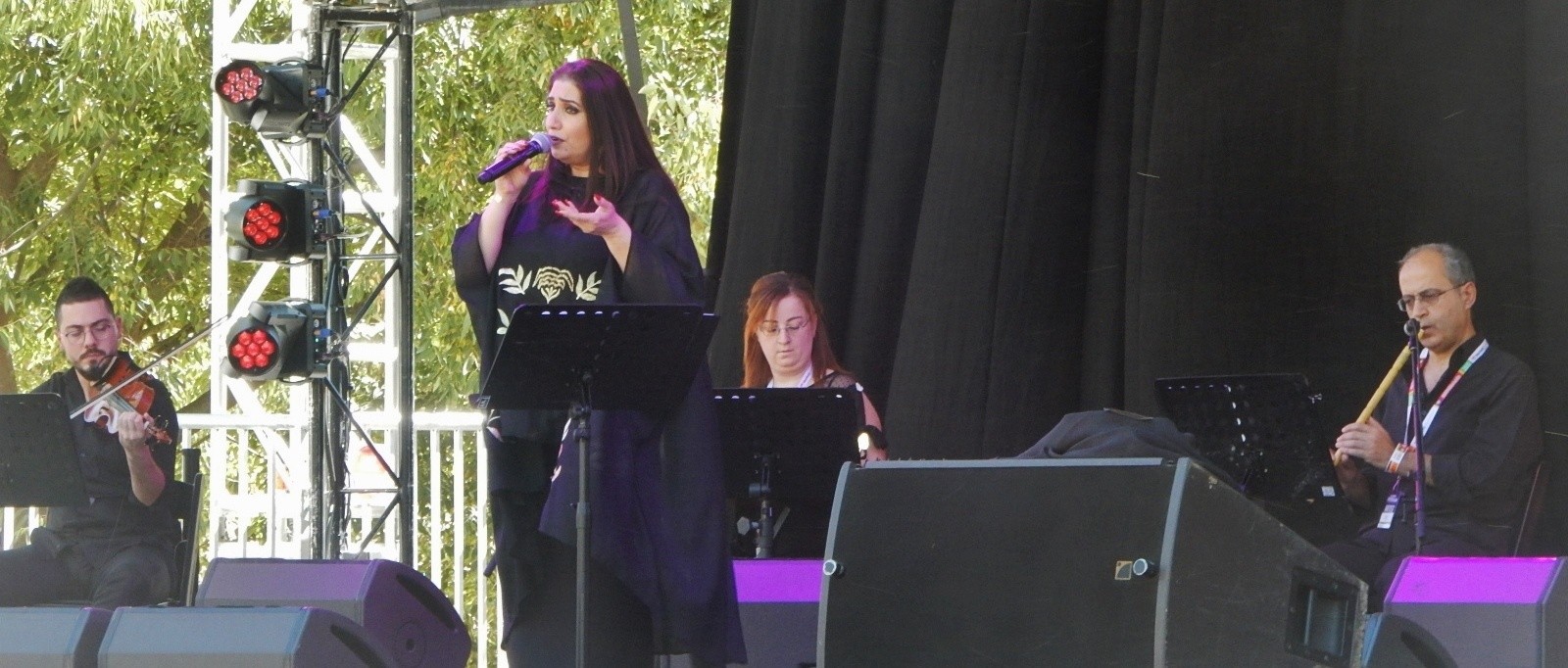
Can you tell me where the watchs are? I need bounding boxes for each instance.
[1383,444,1410,475]
[1312,244,1545,615]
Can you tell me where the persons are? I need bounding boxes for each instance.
[0,276,183,608]
[735,270,886,468]
[450,58,713,668]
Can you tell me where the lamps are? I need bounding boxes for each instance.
[229,300,333,386]
[224,180,336,263]
[215,60,334,139]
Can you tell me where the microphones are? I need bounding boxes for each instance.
[479,131,554,184]
[1403,318,1419,337]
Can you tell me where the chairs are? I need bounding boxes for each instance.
[38,470,203,609]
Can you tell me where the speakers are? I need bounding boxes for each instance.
[1361,553,1568,668]
[193,556,472,667]
[815,454,1369,668]
[96,605,398,667]
[0,605,113,667]
[728,556,826,668]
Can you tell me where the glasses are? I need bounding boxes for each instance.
[1397,281,1468,311]
[759,311,816,337]
[65,324,110,345]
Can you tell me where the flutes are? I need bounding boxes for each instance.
[1333,327,1426,467]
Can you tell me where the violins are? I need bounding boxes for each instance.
[83,354,172,451]
